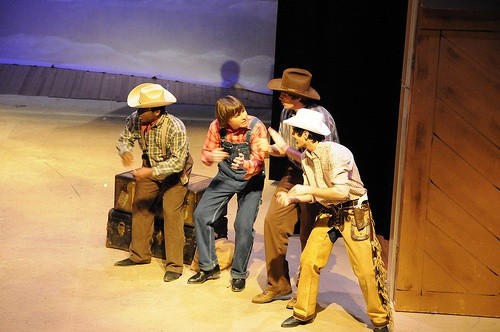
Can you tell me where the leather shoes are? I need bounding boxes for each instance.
[374,325,389,332]
[115,258,149,266]
[231,278,245,292]
[188,264,220,284]
[281,315,315,327]
[286,296,297,309]
[164,271,181,282]
[252,287,292,303]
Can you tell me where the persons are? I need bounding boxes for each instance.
[187,96,268,293]
[113,84,192,282]
[249,68,339,303]
[274,107,391,332]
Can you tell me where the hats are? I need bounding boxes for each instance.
[267,68,320,100]
[127,83,177,108]
[282,107,331,136]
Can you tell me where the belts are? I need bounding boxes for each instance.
[335,198,368,208]
